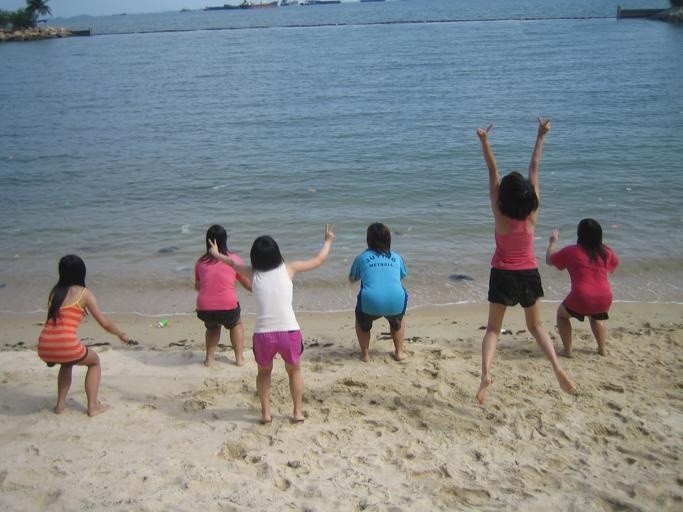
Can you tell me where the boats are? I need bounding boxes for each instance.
[195,0,386,14]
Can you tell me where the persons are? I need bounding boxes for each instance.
[194,224,334,423]
[349,222,408,362]
[37,254,129,417]
